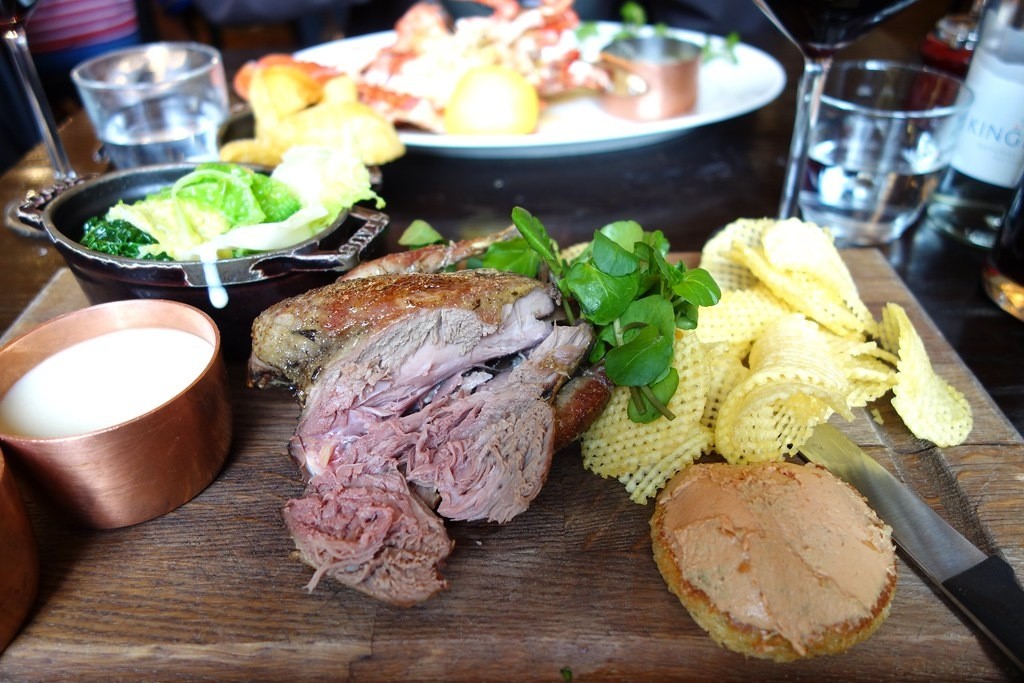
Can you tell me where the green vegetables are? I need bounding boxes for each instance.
[78,161,724,422]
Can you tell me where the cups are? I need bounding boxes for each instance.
[70,39,233,169]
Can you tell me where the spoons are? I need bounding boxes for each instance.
[795,0,920,51]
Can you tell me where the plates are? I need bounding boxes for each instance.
[292,17,785,161]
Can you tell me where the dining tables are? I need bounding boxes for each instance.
[1,44,1024,683]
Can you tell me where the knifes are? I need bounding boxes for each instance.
[797,423,1023,681]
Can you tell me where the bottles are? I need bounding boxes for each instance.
[927,0,1021,248]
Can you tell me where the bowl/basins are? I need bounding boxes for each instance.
[0,299,233,530]
[796,58,975,245]
[4,170,392,336]
[0,450,41,654]
[590,36,705,120]
